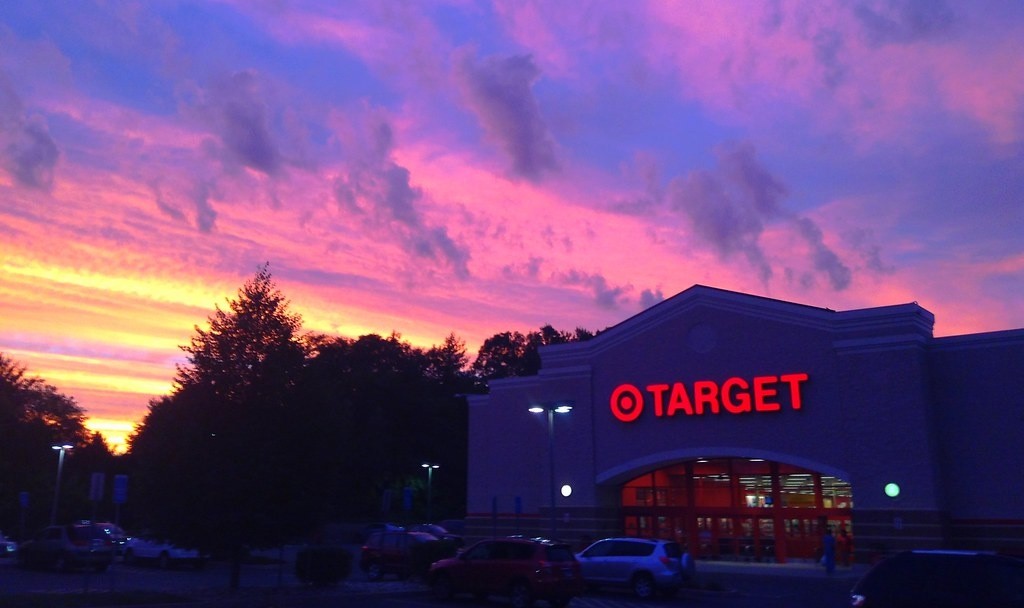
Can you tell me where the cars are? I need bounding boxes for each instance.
[95,522,130,550]
[0,530,19,567]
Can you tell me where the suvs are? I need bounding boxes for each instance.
[573,537,697,599]
[359,531,465,578]
[429,537,584,608]
[20,525,116,572]
[122,531,212,570]
[849,548,1024,608]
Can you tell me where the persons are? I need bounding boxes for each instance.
[836,529,855,567]
[822,529,837,575]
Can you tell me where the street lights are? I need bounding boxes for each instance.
[528,400,577,538]
[50,442,74,524]
[421,463,439,523]
